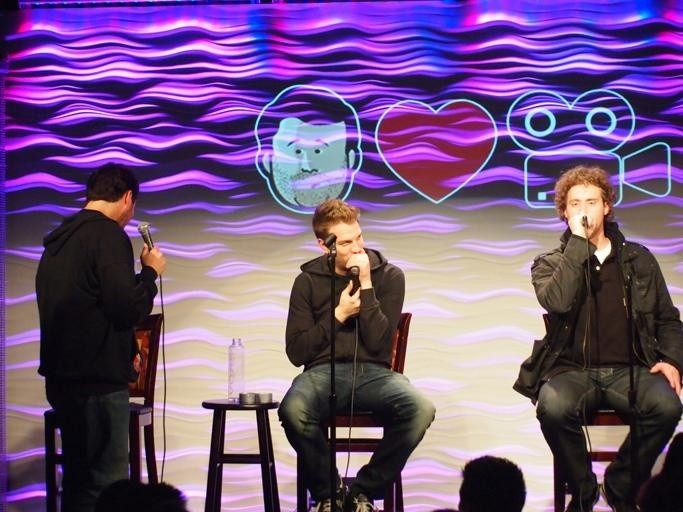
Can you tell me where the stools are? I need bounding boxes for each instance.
[202,399,280,511]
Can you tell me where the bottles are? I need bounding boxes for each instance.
[227,337,246,402]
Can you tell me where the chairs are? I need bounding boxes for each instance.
[43,313,162,511]
[296,312,412,511]
[543,314,651,510]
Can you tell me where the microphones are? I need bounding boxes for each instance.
[350,267,359,277]
[582,215,590,228]
[137,223,154,251]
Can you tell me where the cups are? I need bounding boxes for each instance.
[238,391,257,405]
[255,392,273,405]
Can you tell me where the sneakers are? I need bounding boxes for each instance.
[564,471,640,512]
[314,474,376,512]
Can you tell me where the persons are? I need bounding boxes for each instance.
[458,456,527,510]
[34,162,167,511]
[92,478,188,511]
[276,199,436,511]
[513,166,682,511]
